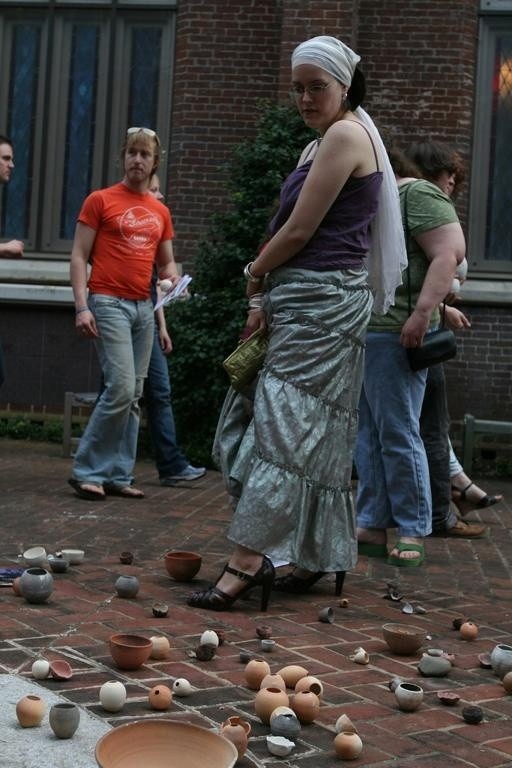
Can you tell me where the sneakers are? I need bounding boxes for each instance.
[162,465,206,483]
[448,520,491,538]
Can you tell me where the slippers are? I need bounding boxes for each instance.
[358,543,387,557]
[106,482,144,498]
[68,478,105,500]
[387,542,425,567]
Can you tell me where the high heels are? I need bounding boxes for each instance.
[450,482,504,515]
[274,566,346,595]
[187,555,275,612]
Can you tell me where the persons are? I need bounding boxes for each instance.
[144,172,205,485]
[68,125,178,499]
[353,137,503,567]
[0,135,25,257]
[186,35,410,612]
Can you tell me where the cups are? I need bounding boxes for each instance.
[318,606,335,623]
[262,640,275,652]
[240,652,253,664]
[267,736,295,756]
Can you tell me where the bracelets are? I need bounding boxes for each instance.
[242,260,254,282]
[74,308,87,312]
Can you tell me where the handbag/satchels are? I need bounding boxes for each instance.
[222,328,269,388]
[407,328,456,372]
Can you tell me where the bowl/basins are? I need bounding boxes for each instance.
[23,546,47,567]
[110,634,152,669]
[63,549,85,564]
[95,719,238,768]
[335,713,358,734]
[166,552,201,580]
[382,623,427,655]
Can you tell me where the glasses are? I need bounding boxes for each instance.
[289,83,332,94]
[127,127,160,148]
